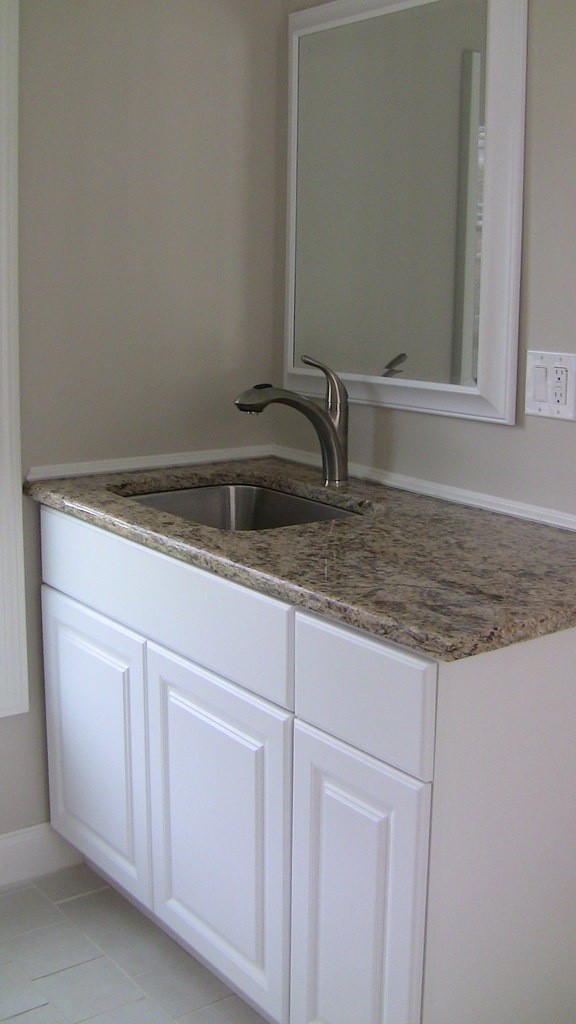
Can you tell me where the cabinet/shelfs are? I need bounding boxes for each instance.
[39,501,291,1024]
[290,609,576,1024]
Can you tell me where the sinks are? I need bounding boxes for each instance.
[107,472,382,533]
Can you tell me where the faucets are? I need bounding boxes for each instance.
[234,354,350,486]
[383,351,410,378]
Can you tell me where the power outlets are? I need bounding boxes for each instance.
[524,350,576,422]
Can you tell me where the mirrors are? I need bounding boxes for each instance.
[285,0,528,424]
